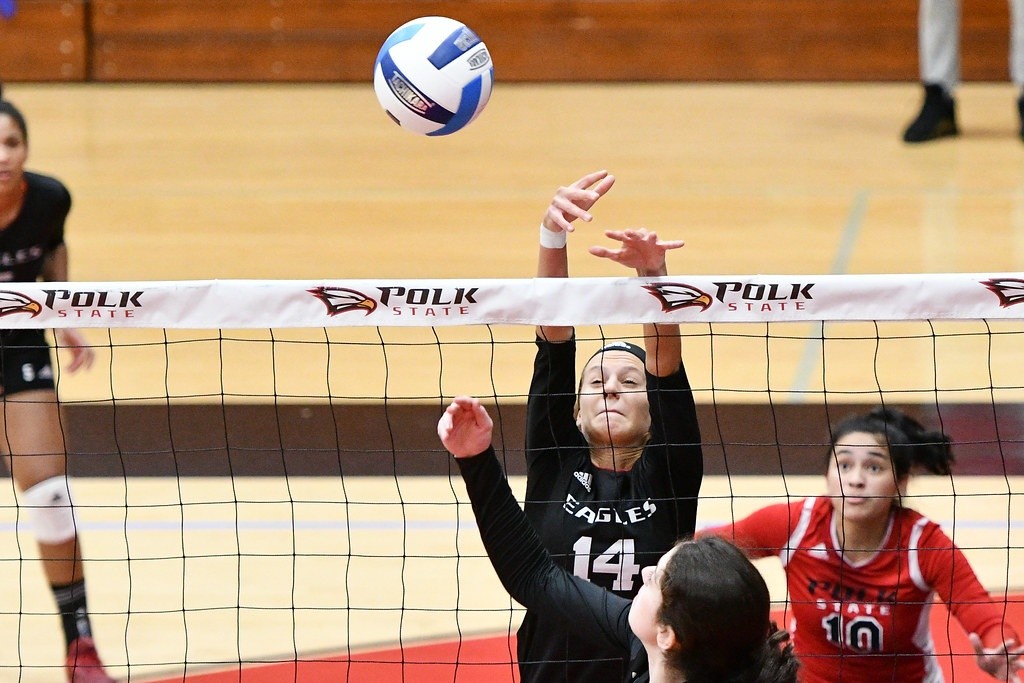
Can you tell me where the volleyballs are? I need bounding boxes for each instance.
[373,15,495,137]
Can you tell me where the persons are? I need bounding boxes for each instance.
[903,0,1024,142]
[439,169,1024,683]
[0,102,113,683]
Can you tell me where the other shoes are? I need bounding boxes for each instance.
[65,637,115,683]
[902,87,958,143]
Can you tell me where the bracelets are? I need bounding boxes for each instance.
[540,222,566,249]
